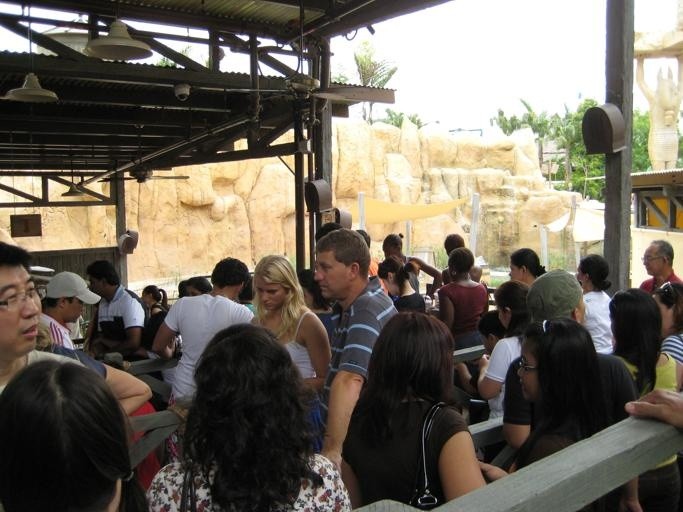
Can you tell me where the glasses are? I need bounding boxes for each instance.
[0,285,47,311]
[519,357,539,371]
[660,282,675,297]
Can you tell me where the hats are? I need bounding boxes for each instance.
[46,271,101,304]
[526,269,581,319]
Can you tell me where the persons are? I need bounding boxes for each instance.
[635,53,682,171]
[0,223,682,512]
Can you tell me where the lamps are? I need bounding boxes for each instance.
[0,2,59,103]
[83,2,153,61]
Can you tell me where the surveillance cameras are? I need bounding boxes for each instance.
[175,86,190,100]
